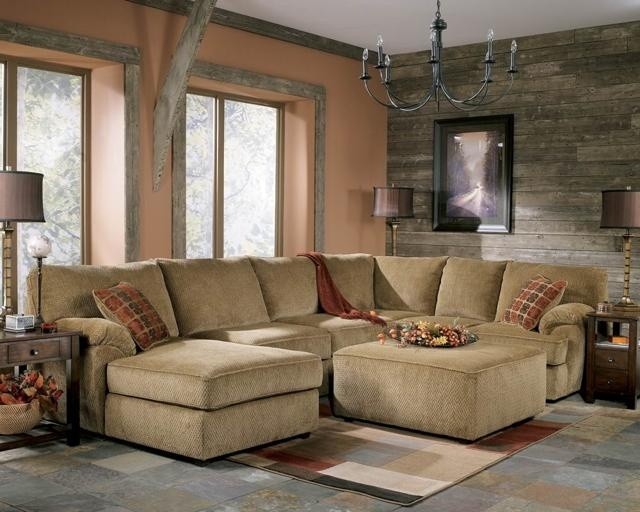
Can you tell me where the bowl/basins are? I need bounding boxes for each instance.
[598,303,613,313]
[40,322,57,332]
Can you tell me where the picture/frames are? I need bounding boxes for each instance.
[433,114,510,233]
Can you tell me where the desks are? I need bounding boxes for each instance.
[0,329,92,454]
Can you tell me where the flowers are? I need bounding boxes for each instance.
[0,372,64,413]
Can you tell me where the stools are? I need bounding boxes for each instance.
[104,340,323,467]
[333,340,547,441]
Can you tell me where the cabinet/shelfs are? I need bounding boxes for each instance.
[583,308,640,410]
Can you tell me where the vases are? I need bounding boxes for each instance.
[0,403,41,435]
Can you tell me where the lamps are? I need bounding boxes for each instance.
[0,170,46,320]
[600,190,640,310]
[28,235,51,327]
[371,186,415,256]
[359,0,518,112]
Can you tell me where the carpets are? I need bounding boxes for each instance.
[231,417,572,505]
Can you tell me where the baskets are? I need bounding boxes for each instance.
[0,399,41,435]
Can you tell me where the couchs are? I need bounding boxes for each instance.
[28,253,610,434]
